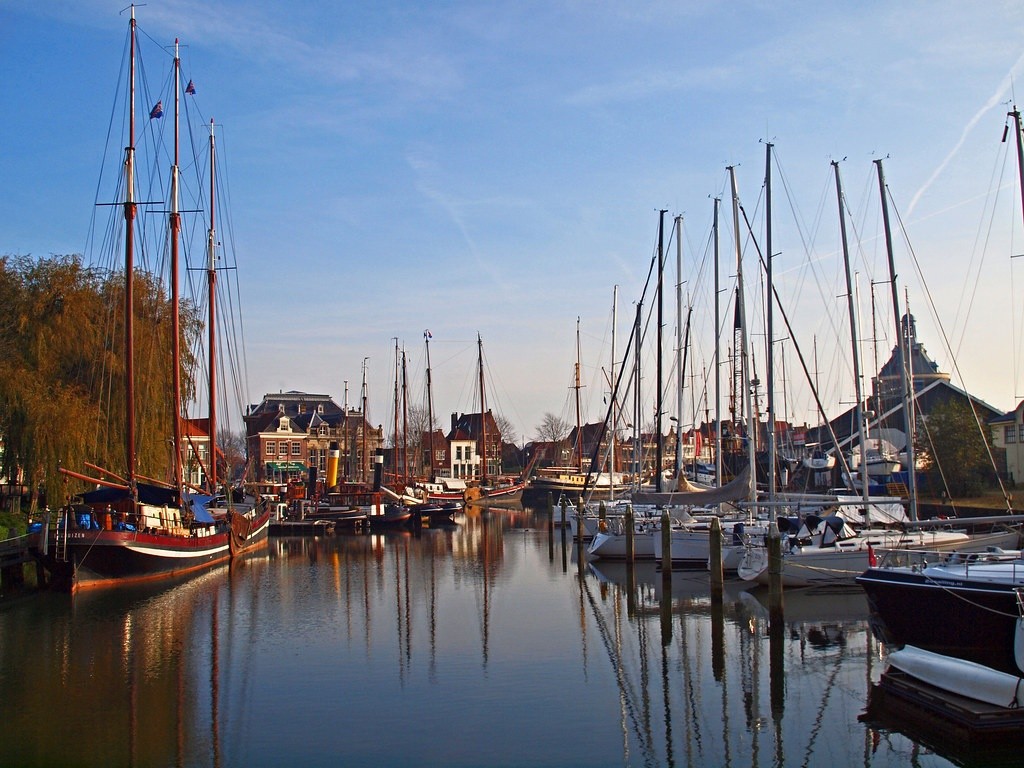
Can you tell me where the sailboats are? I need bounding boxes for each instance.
[555,101,1024,733]
[27,1,270,596]
[243,316,637,539]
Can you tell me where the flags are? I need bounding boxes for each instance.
[149,100,163,119]
[424,331,432,338]
[185,80,196,96]
